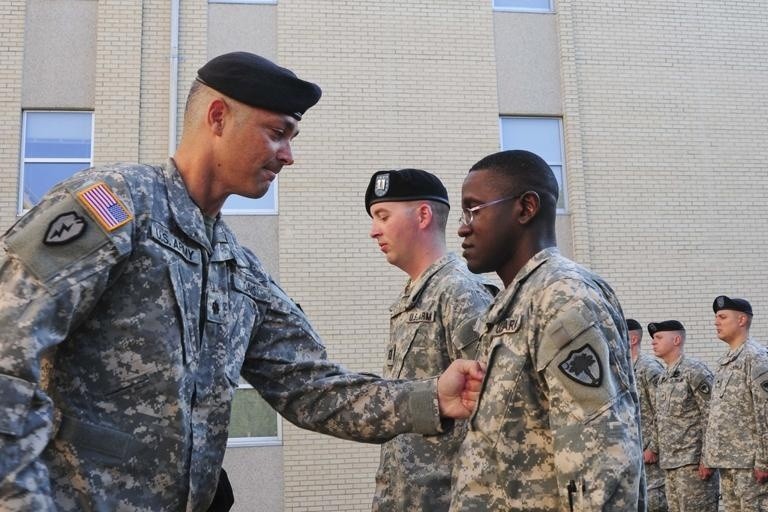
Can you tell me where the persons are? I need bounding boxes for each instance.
[363,168,495,511]
[444,149,644,512]
[646,320,720,512]
[703,295,767,512]
[625,317,668,512]
[1,50,487,510]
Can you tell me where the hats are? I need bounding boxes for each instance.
[626,319,642,331]
[648,320,685,338]
[197,52,323,120]
[365,168,451,219]
[712,296,753,317]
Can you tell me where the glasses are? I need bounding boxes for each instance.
[458,194,521,225]
